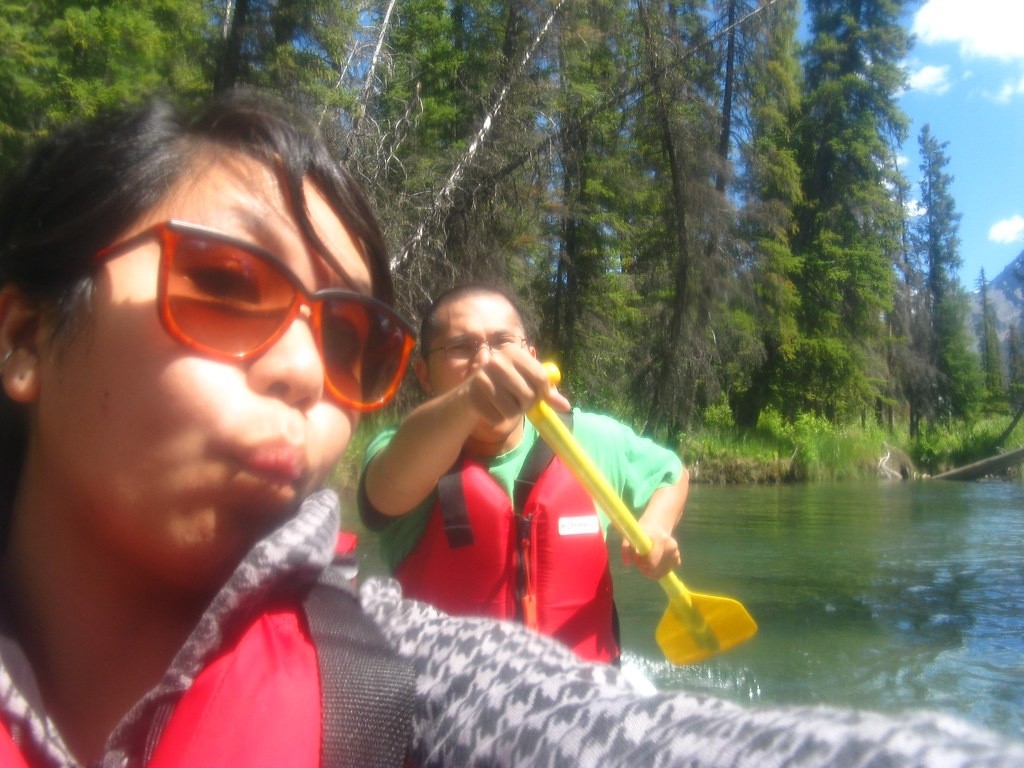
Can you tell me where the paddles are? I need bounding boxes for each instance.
[524,360,758,665]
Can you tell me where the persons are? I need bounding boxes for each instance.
[0,89,1024,768]
[355,282,689,673]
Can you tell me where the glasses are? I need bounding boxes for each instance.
[29,220,417,411]
[424,335,526,362]
